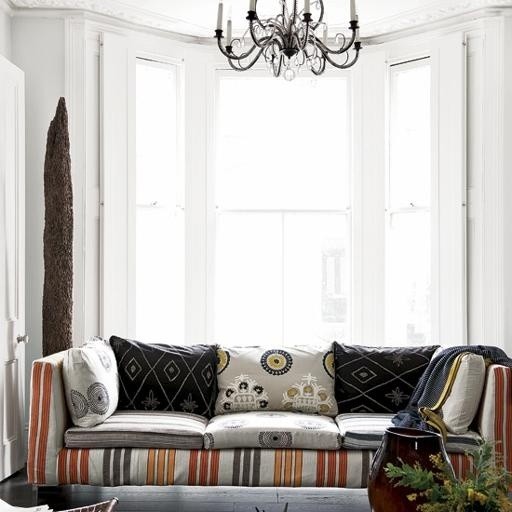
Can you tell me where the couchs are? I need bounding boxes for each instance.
[27,348,512,488]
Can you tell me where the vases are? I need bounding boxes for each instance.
[368,427,456,512]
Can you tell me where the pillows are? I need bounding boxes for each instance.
[214,345,339,418]
[63,337,119,429]
[332,341,442,414]
[430,344,484,434]
[109,337,219,421]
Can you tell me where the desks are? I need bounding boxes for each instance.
[0,486,512,512]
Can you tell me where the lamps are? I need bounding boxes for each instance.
[214,0,362,81]
